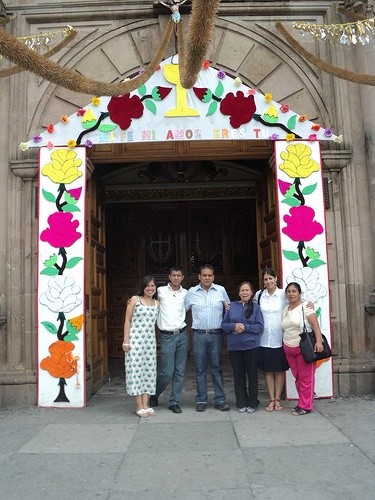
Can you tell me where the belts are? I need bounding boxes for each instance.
[192,329,223,335]
[160,326,187,335]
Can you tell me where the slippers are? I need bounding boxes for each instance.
[136,409,149,418]
[146,408,156,416]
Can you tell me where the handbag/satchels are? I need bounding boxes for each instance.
[299,332,332,364]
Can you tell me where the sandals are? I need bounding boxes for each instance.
[291,406,300,412]
[275,399,284,411]
[266,400,275,411]
[292,408,311,416]
[239,406,247,412]
[247,407,256,413]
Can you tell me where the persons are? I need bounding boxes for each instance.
[222,280,264,413]
[252,269,314,411]
[122,275,160,418]
[282,281,333,416]
[185,263,230,412]
[150,266,189,413]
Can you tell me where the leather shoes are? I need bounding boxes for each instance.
[215,403,231,410]
[196,404,206,411]
[150,396,158,406]
[169,404,182,413]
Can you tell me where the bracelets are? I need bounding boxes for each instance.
[122,343,130,345]
